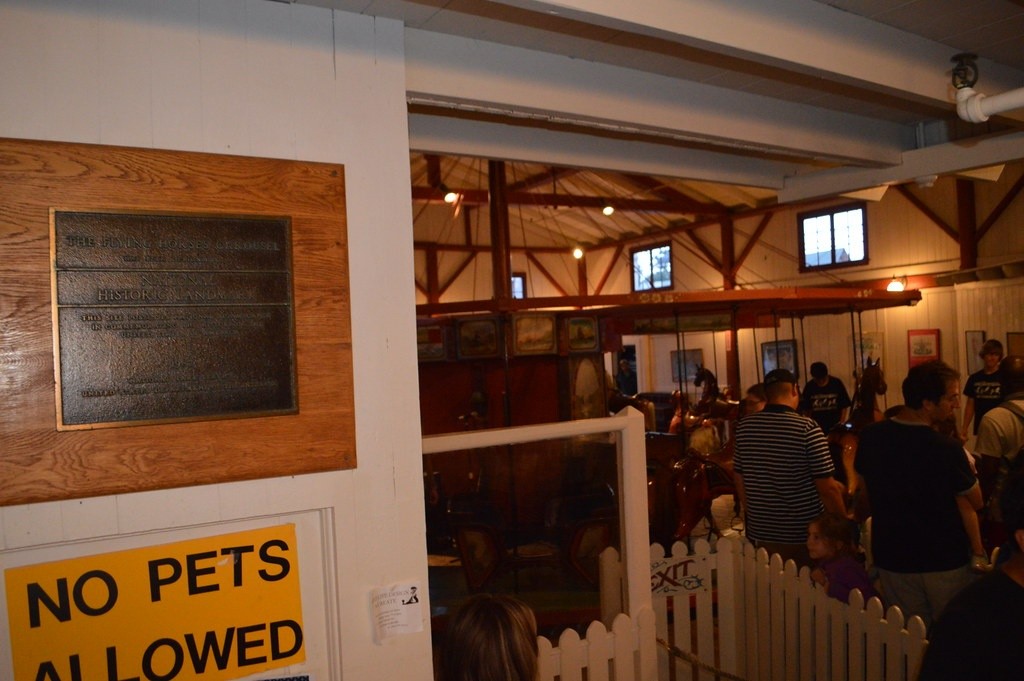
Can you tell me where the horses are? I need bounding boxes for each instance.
[693,364,740,424]
[829,357,887,462]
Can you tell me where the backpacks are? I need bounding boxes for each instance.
[997,402,1024,526]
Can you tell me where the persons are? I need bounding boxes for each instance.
[732,338,1024,681]
[438,593,540,681]
[615,359,637,395]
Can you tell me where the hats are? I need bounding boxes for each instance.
[810,361,827,377]
[619,359,629,364]
[764,369,805,402]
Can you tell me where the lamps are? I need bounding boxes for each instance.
[437,183,457,203]
[600,197,614,216]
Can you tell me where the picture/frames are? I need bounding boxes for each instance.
[964,330,987,376]
[761,339,800,382]
[1006,331,1024,357]
[670,349,704,383]
[846,331,884,378]
[905,329,942,370]
[417,311,599,360]
